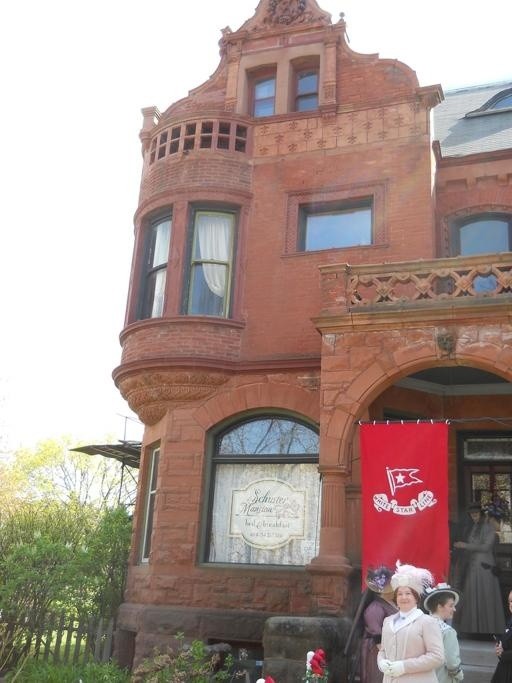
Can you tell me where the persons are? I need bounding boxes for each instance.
[485,590,512,683]
[351,566,394,682]
[421,581,466,682]
[450,499,498,641]
[375,559,444,682]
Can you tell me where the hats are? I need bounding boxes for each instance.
[363,559,458,613]
[465,502,482,513]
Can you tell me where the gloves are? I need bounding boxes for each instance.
[377,659,406,678]
